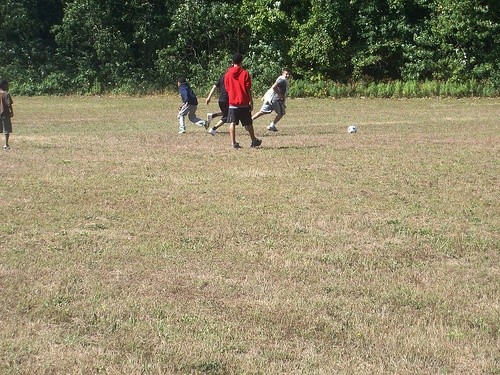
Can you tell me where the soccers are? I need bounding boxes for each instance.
[348,126,358,134]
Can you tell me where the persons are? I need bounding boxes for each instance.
[205,67,229,136]
[0,80,14,149]
[177,77,209,134]
[252,68,290,132]
[224,54,262,148]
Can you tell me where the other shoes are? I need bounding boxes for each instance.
[250,138,263,147]
[267,124,279,131]
[177,130,186,134]
[207,113,214,126]
[231,142,243,148]
[3,145,11,150]
[208,128,218,136]
[203,120,209,130]
